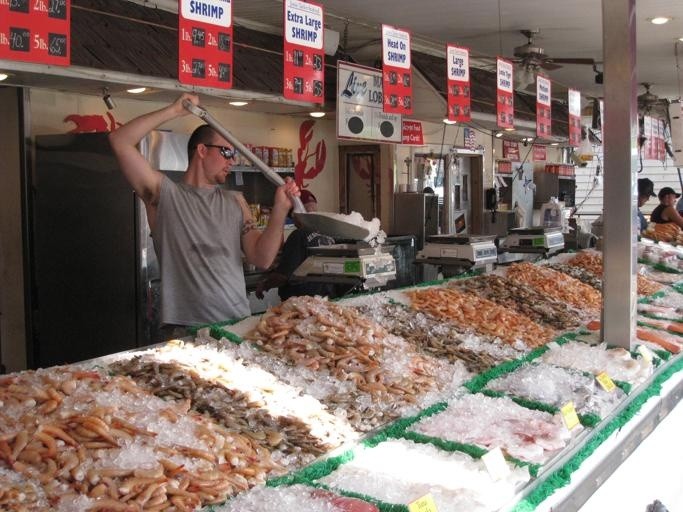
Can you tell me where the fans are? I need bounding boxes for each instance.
[468,28,596,73]
[636,82,679,109]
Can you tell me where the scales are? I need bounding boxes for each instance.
[414,234,498,266]
[496,225,565,254]
[293,244,397,284]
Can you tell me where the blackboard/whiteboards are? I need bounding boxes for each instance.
[335,59,403,145]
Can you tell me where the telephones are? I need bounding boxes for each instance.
[486,187,497,224]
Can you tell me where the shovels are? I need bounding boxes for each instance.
[183,99,370,242]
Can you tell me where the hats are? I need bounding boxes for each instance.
[638,178,656,196]
[658,187,681,198]
[300,190,317,204]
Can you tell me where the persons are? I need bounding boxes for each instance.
[109,93,300,343]
[637,178,657,234]
[285,189,317,225]
[650,187,683,229]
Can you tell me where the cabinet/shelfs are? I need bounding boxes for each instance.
[228,164,297,231]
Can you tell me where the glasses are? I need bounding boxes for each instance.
[195,145,235,160]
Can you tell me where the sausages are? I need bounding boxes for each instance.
[647,223,681,241]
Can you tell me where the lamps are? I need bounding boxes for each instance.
[514,57,550,86]
